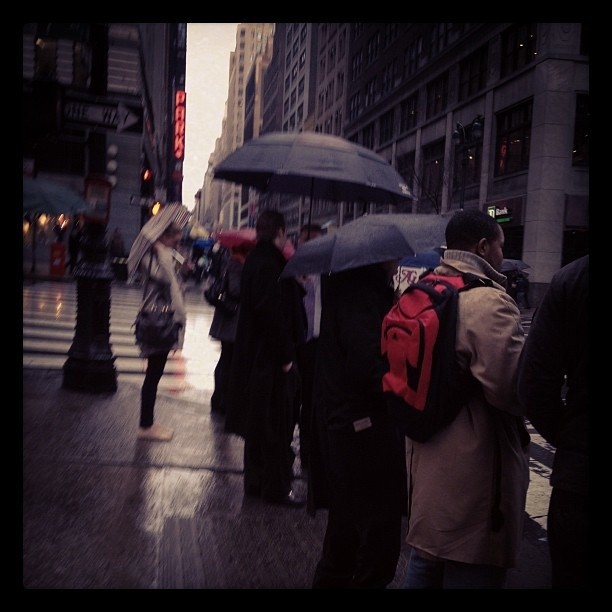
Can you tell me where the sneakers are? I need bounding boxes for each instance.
[137,430,173,443]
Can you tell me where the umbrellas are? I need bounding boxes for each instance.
[24,176,86,271]
[276,211,449,281]
[217,229,295,262]
[214,130,412,241]
[500,258,529,274]
[124,203,186,284]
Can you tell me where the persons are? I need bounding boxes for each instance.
[203,240,255,417]
[132,220,185,443]
[506,272,530,313]
[378,210,525,594]
[515,254,601,590]
[286,224,325,339]
[108,228,127,280]
[312,254,401,590]
[226,209,310,507]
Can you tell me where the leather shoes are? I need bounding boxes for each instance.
[268,491,307,508]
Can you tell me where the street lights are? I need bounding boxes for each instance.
[452,115,481,208]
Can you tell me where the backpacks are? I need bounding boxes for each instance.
[382,264,508,445]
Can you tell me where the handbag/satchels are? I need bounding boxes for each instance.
[130,278,183,349]
[204,260,240,319]
